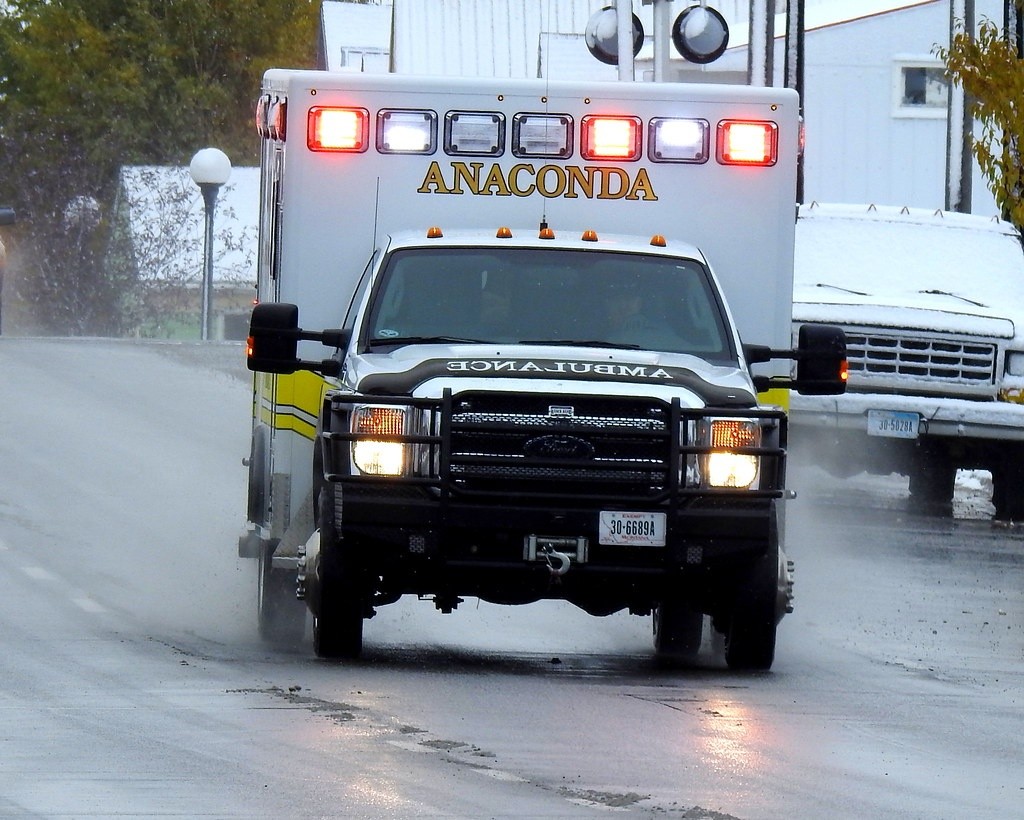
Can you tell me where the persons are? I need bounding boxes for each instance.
[446,278,517,340]
[585,274,676,343]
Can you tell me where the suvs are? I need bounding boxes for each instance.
[795,200,1024,521]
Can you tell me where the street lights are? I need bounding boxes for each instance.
[189,147,232,340]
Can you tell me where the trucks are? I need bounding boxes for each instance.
[238,67,800,675]
